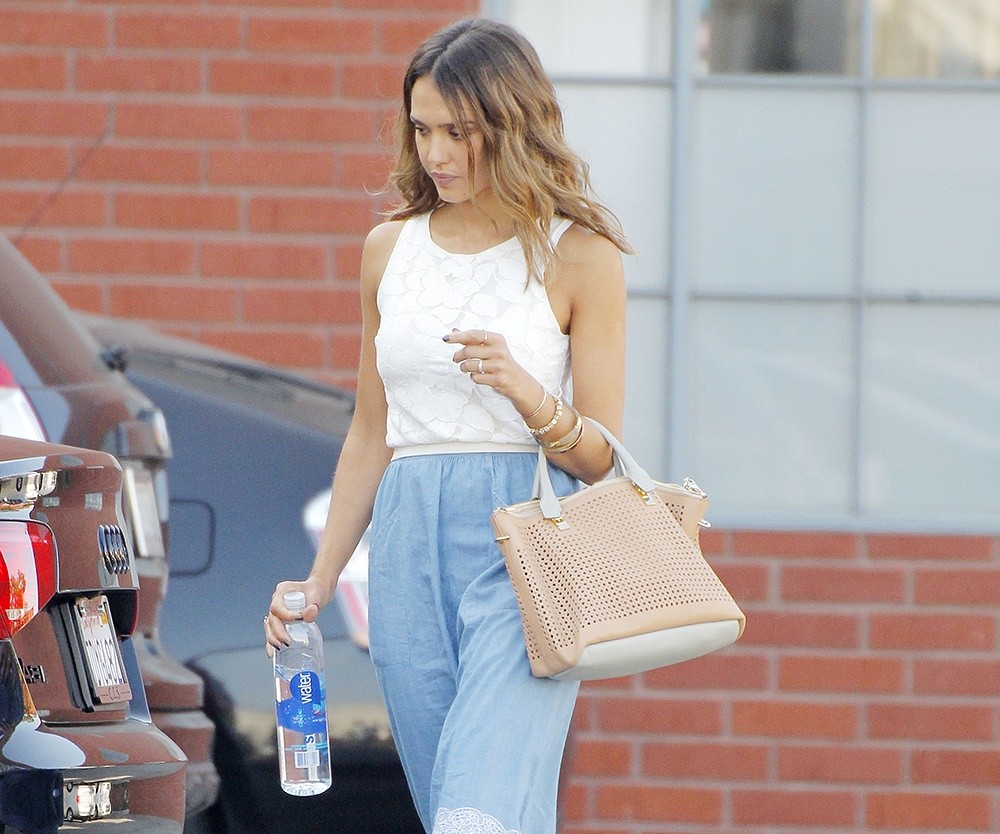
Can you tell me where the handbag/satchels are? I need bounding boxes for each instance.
[491,414,746,682]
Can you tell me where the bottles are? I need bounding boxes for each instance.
[270,591,331,797]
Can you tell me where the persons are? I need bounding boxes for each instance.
[262,15,628,834]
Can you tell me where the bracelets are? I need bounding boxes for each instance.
[522,385,585,453]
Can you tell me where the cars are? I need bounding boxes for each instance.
[0,305,428,834]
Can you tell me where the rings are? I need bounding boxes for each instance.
[478,360,486,374]
[480,329,488,345]
[263,615,270,624]
